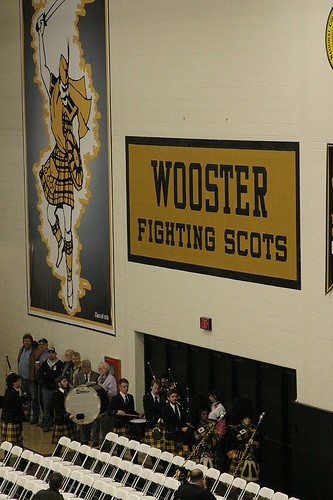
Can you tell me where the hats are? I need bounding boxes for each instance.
[38,338,48,343]
[232,396,253,426]
[48,347,55,352]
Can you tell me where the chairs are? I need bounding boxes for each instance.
[0,432,299,500]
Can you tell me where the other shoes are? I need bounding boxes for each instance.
[21,417,31,422]
[30,419,39,425]
[19,461,35,469]
[43,425,50,432]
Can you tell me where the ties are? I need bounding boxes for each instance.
[154,396,159,412]
[175,404,179,421]
[125,395,128,405]
[84,374,88,382]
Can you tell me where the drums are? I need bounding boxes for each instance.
[65,381,110,425]
[129,418,147,439]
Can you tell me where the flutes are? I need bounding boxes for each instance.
[19,386,37,404]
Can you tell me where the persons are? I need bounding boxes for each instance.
[0,372,36,470]
[162,388,189,476]
[61,348,75,379]
[142,377,170,471]
[207,391,226,442]
[107,377,145,465]
[225,412,261,500]
[37,346,64,432]
[36,337,51,428]
[50,375,75,446]
[173,468,218,500]
[17,332,43,425]
[90,360,118,450]
[182,405,222,489]
[73,359,100,448]
